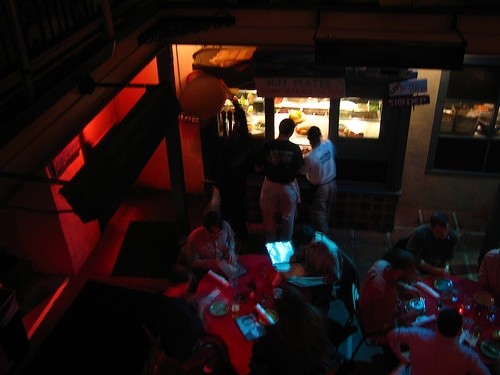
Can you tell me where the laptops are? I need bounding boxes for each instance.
[265,241,296,273]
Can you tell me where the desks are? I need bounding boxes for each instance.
[402,276,500,368]
[194,255,311,375]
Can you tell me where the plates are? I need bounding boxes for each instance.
[433,276,454,291]
[405,300,426,316]
[480,338,500,359]
[209,301,229,316]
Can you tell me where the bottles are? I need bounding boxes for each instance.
[230,293,240,318]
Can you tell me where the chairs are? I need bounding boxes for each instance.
[335,283,387,360]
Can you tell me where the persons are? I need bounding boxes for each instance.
[257,119,306,241]
[141,212,500,375]
[299,127,339,235]
